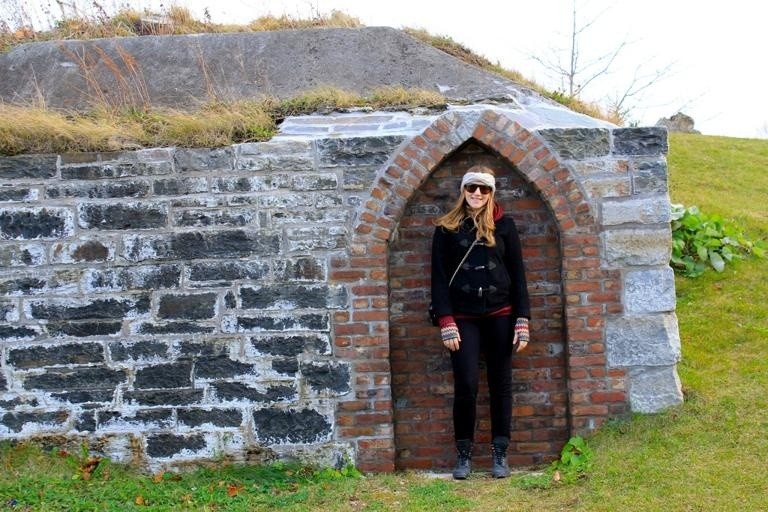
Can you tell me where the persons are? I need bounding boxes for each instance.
[429,163,531,481]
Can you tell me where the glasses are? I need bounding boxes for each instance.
[464,183,492,194]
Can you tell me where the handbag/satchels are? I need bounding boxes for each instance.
[425,301,438,327]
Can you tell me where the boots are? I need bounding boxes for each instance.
[490,437,511,478]
[453,438,475,478]
